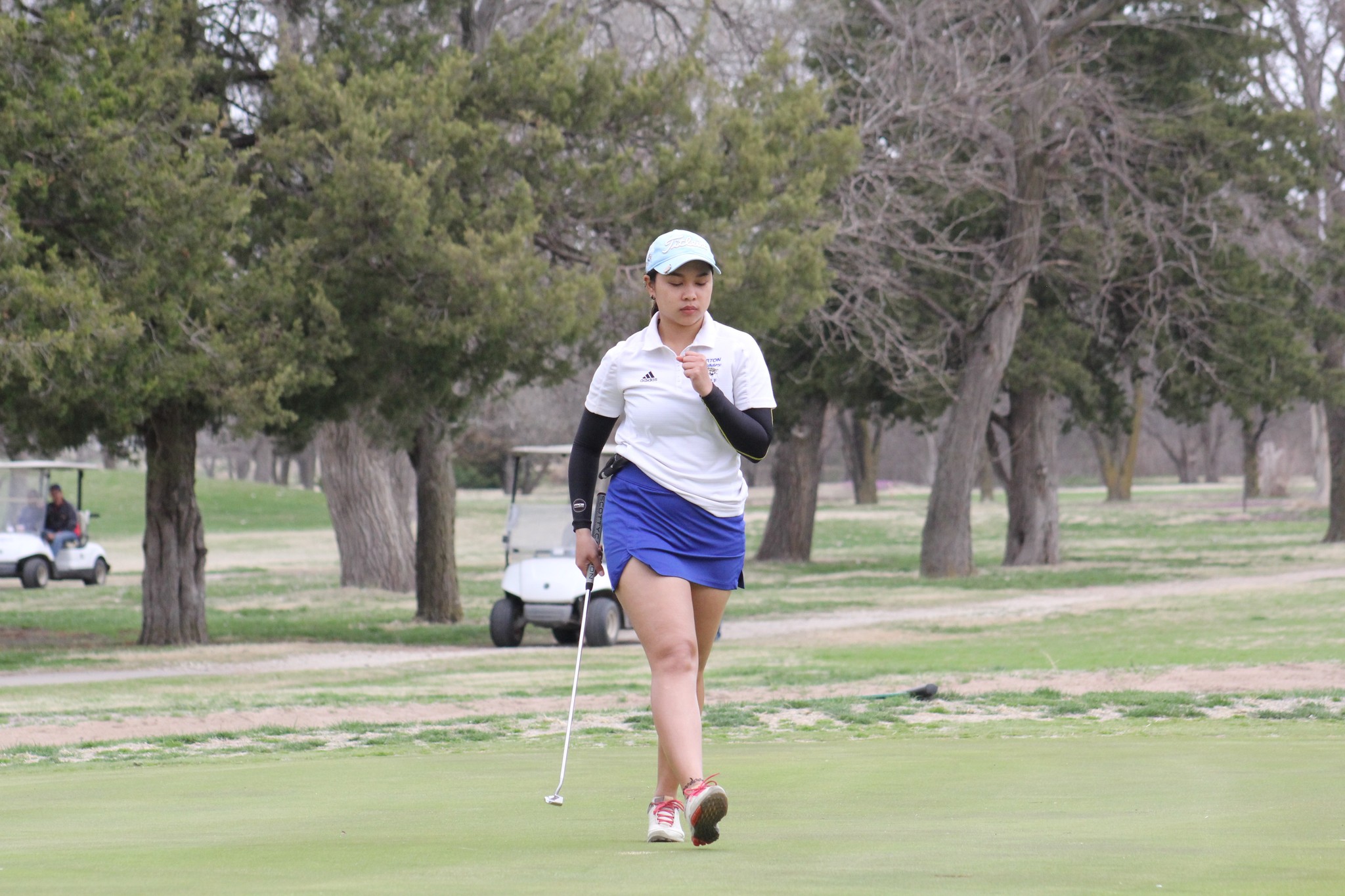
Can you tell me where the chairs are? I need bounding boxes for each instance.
[62,510,91,548]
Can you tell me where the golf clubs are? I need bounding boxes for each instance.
[544,492,606,806]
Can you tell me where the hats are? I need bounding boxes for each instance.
[645,229,721,275]
[50,485,61,492]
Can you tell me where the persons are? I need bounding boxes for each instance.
[6,484,80,558]
[568,230,776,846]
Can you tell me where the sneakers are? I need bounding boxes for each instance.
[684,773,728,846]
[647,796,685,842]
